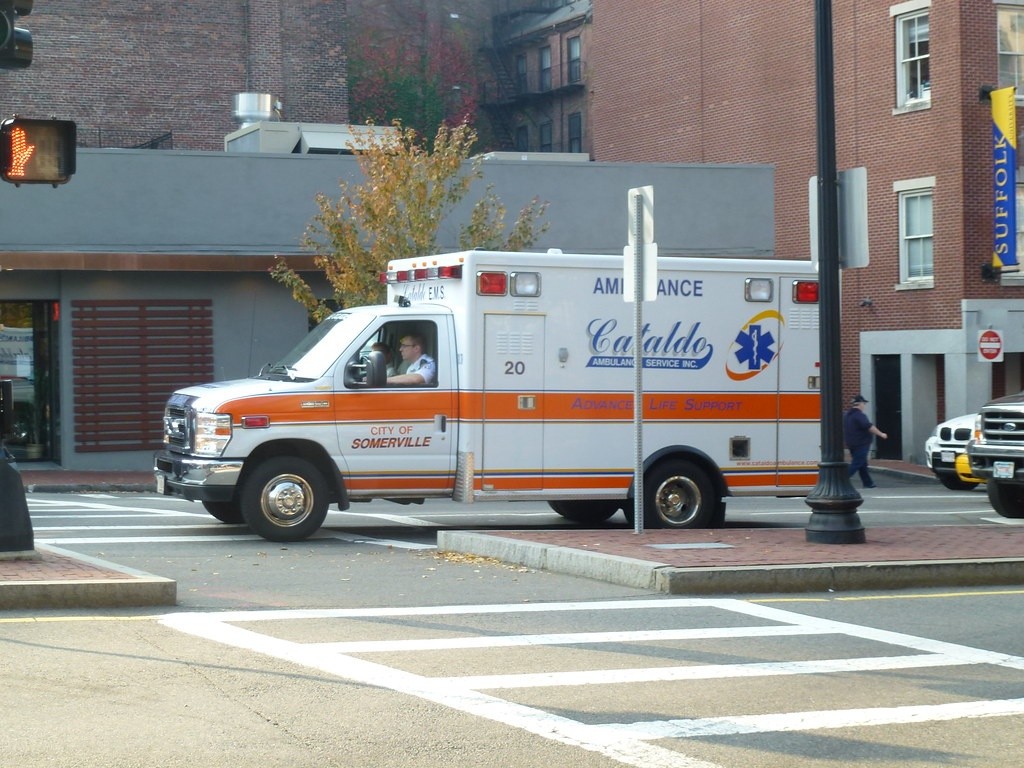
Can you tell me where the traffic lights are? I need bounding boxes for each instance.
[1,1,77,189]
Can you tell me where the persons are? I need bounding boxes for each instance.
[841,393,887,489]
[369,335,437,386]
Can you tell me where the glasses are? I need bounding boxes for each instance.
[400,344,415,348]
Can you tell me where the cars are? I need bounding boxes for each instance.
[924,414,983,490]
[967,391,1024,519]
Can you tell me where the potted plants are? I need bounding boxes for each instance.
[13,365,50,458]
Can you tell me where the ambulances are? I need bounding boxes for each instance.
[153,249,844,544]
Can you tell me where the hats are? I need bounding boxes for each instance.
[851,396,868,403]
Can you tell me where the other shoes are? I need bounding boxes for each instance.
[868,484,877,488]
